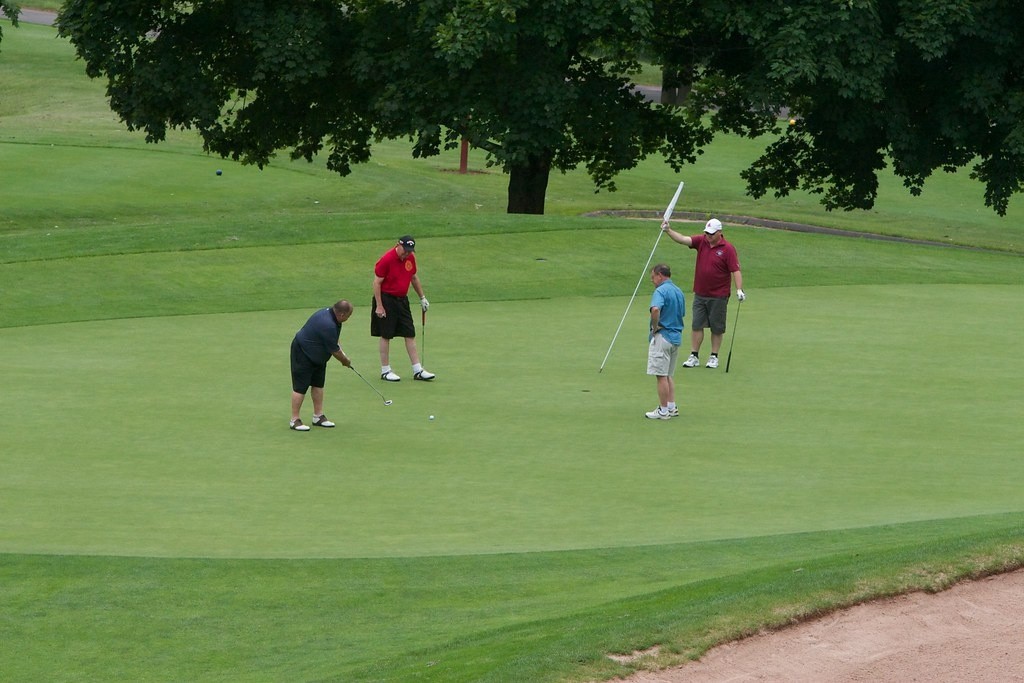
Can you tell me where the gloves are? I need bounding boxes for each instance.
[737,289,745,303]
[420,296,429,312]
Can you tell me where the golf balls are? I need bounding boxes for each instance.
[430,415,434,420]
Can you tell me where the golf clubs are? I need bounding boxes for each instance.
[422,307,426,369]
[725,297,744,373]
[349,365,393,406]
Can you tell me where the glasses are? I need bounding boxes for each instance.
[705,232,715,235]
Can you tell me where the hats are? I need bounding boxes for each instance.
[703,219,722,234]
[398,235,416,253]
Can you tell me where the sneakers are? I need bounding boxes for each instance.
[706,356,719,368]
[312,415,335,427]
[290,418,310,431]
[413,368,435,380]
[381,369,400,381]
[683,355,699,367]
[645,405,679,420]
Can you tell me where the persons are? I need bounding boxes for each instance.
[290,299,354,431]
[661,219,746,368]
[645,264,685,420]
[371,235,435,382]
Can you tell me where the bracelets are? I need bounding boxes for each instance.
[419,295,425,300]
